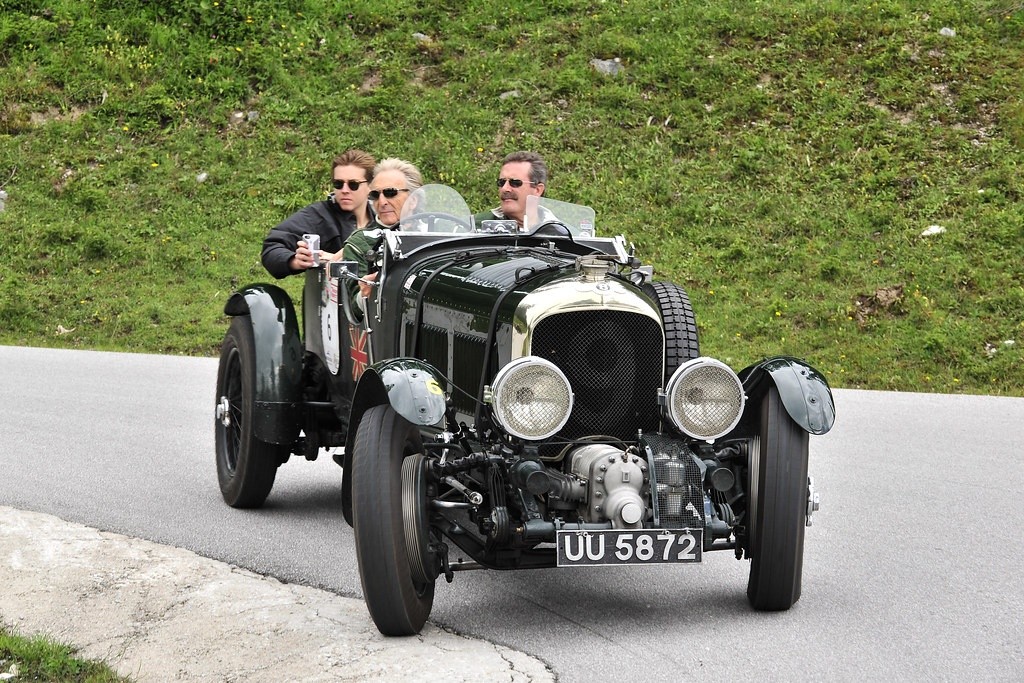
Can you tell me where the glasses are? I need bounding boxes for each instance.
[331,179,368,191]
[496,178,538,188]
[367,187,409,200]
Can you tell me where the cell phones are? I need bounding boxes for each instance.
[303,234,320,268]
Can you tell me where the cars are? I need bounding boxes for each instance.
[214,185,837,636]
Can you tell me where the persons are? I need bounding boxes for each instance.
[473,151,582,236]
[261,149,425,323]
